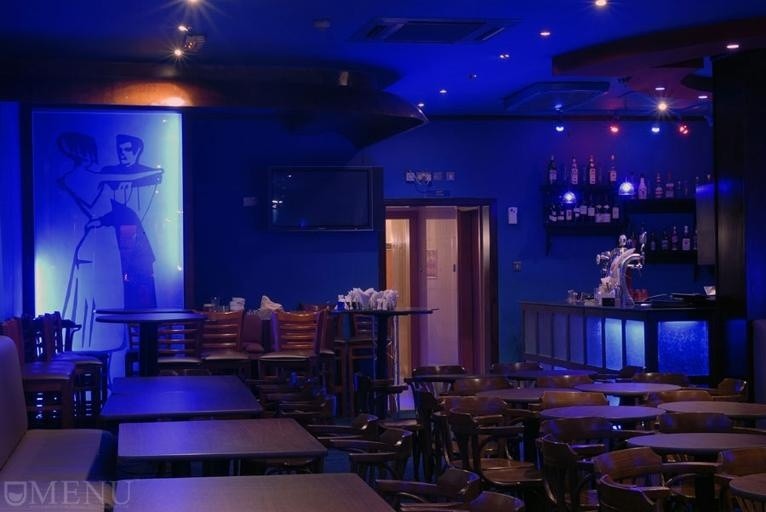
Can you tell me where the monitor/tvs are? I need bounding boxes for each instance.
[271,166,373,232]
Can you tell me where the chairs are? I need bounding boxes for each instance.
[0,290,766,512]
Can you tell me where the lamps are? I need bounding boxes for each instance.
[561,188,577,205]
[171,0,199,60]
[618,178,636,197]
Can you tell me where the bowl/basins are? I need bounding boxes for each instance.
[228,297,245,311]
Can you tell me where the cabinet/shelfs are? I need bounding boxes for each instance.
[625,197,692,272]
[542,179,625,257]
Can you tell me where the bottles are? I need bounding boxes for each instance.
[547,195,618,225]
[548,148,618,191]
[629,219,699,252]
[626,167,710,201]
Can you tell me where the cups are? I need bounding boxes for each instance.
[210,295,220,311]
